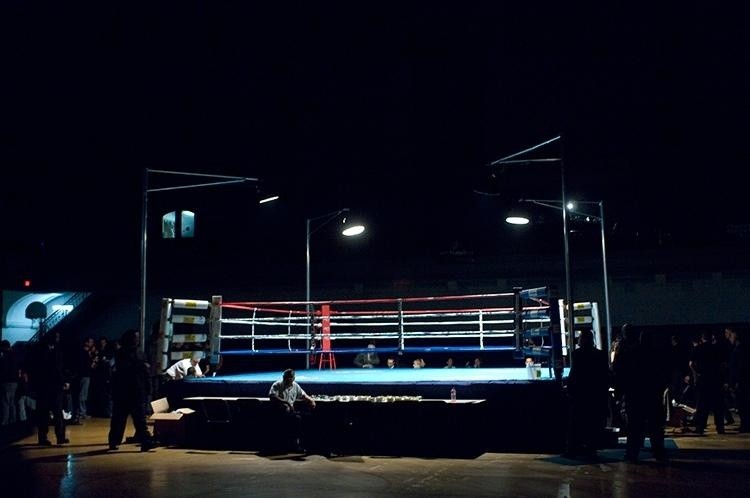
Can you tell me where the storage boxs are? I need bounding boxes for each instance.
[148,395,198,433]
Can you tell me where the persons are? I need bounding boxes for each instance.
[559,321,750,469]
[352,343,485,369]
[266,368,346,458]
[523,354,543,369]
[0,330,226,452]
